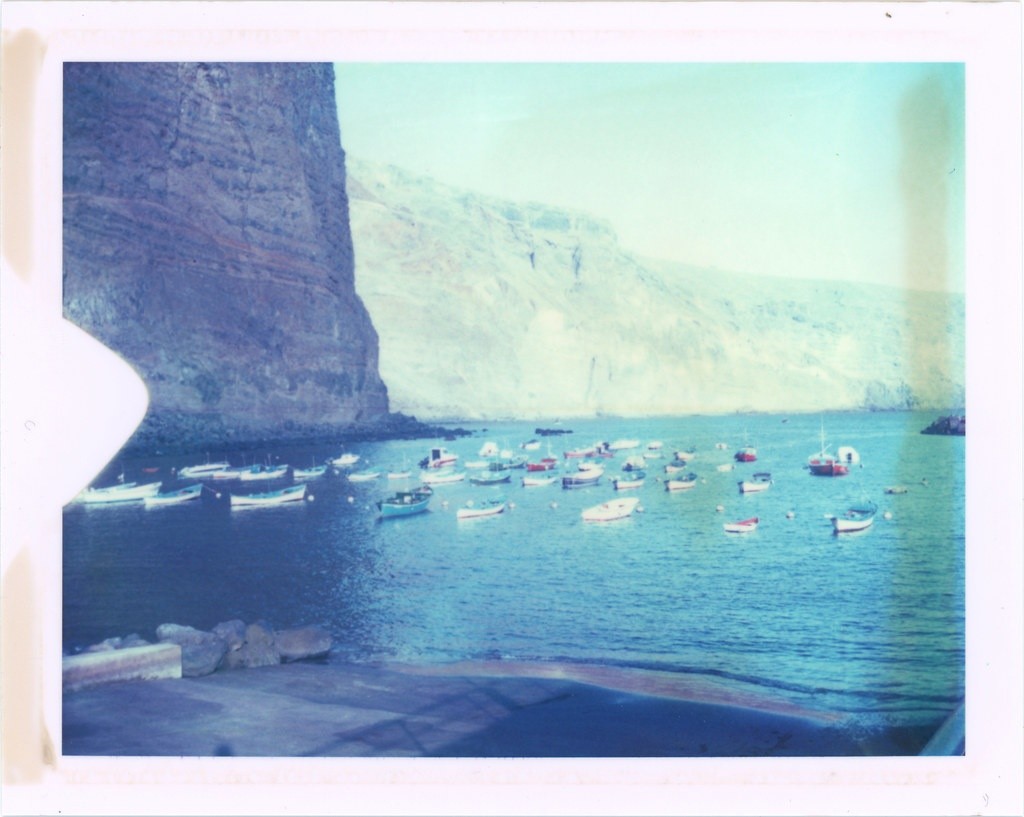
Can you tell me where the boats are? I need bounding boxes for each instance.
[831,500,878,532]
[145,483,203,505]
[581,496,637,520]
[675,446,697,459]
[723,517,759,533]
[735,445,757,462]
[457,497,506,519]
[180,452,288,483]
[230,484,307,506]
[328,438,559,488]
[377,485,433,518]
[665,472,696,489]
[562,438,663,488]
[808,453,847,476]
[292,466,327,479]
[613,471,646,490]
[622,454,645,471]
[665,459,687,472]
[737,471,772,493]
[74,481,163,504]
